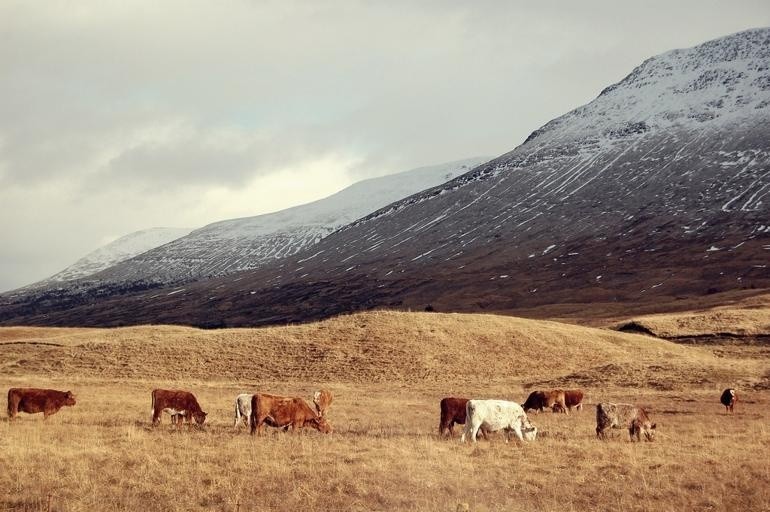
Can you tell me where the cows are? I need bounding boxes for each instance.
[8,388,77,421]
[151,388,208,428]
[596,403,657,442]
[438,397,489,440]
[720,388,739,412]
[460,399,537,444]
[521,389,584,414]
[232,387,338,434]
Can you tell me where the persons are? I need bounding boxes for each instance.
[425,303,434,313]
